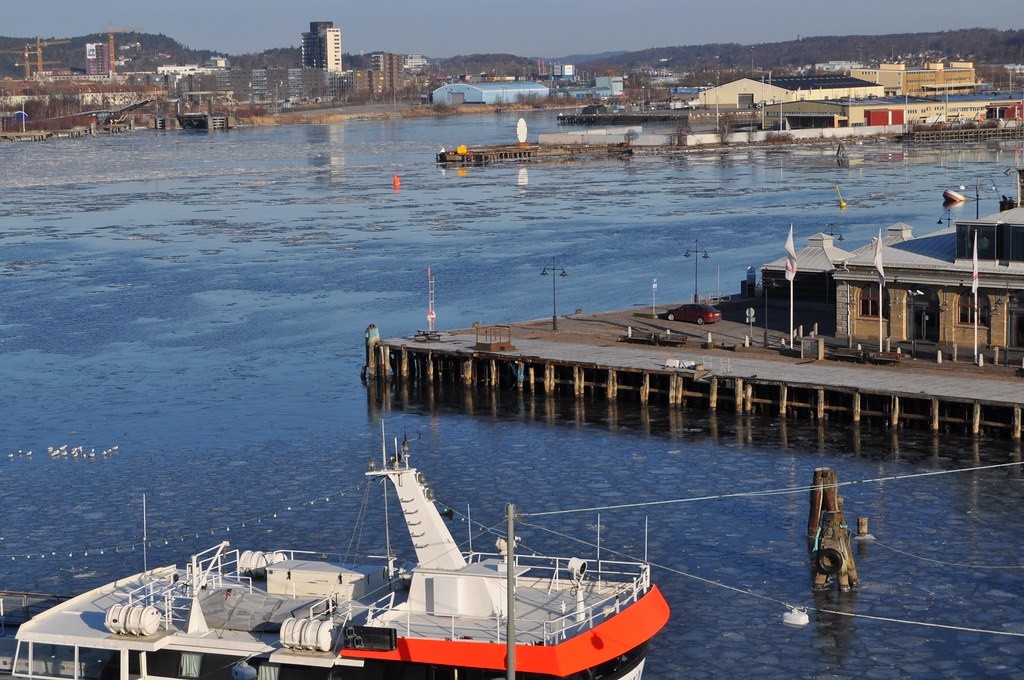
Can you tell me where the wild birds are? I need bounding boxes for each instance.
[7,444,118,459]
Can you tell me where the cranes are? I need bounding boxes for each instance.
[0,39,72,80]
[98,20,146,74]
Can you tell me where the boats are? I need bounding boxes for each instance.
[0,420,672,680]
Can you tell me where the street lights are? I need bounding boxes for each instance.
[822,223,844,246]
[539,257,570,330]
[959,175,996,220]
[682,239,710,304]
[937,208,958,228]
[907,289,925,357]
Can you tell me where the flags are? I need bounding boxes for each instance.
[873,229,886,287]
[971,232,979,294]
[783,225,798,281]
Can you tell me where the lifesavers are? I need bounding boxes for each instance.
[427,310,436,327]
[815,547,843,575]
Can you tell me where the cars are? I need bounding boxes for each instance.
[664,303,723,325]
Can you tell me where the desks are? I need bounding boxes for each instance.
[417,330,437,340]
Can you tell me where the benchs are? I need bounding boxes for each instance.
[628,331,652,345]
[871,352,904,367]
[414,334,442,342]
[654,333,687,348]
[833,347,863,362]
